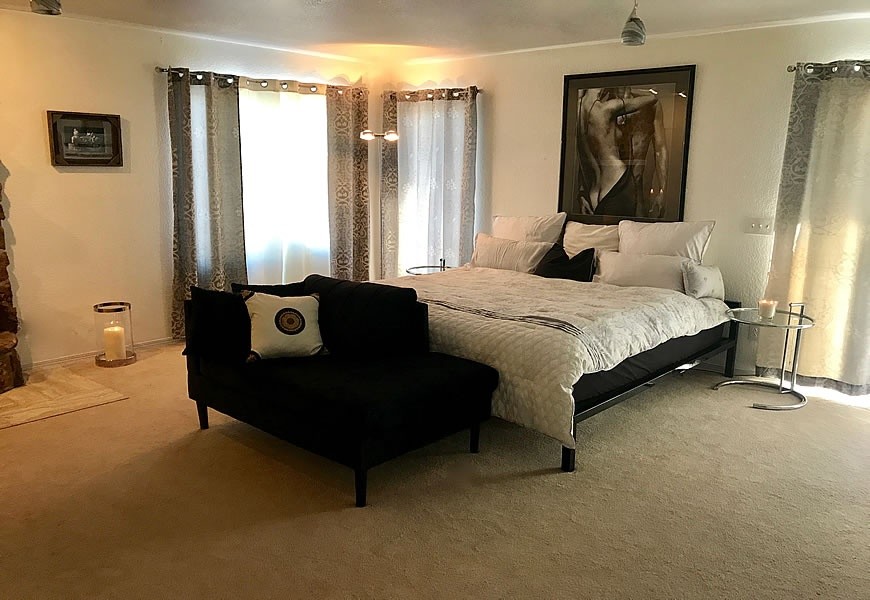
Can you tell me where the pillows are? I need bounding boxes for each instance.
[242,289,328,362]
[535,244,595,282]
[492,212,567,243]
[680,261,725,301]
[619,219,717,263]
[469,231,553,273]
[594,247,692,292]
[563,220,619,257]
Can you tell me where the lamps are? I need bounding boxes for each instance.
[621,1,646,45]
[360,128,398,142]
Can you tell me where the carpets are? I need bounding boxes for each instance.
[0,364,129,429]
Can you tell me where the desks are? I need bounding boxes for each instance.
[714,302,817,410]
[406,257,453,276]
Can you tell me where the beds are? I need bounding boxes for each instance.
[361,264,742,471]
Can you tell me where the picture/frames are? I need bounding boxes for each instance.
[557,65,696,226]
[47,110,123,167]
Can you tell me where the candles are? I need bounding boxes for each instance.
[104,327,126,361]
[758,300,778,317]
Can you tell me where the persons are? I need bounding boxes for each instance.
[575,88,657,219]
[577,86,669,220]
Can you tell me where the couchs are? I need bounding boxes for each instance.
[184,272,500,507]
